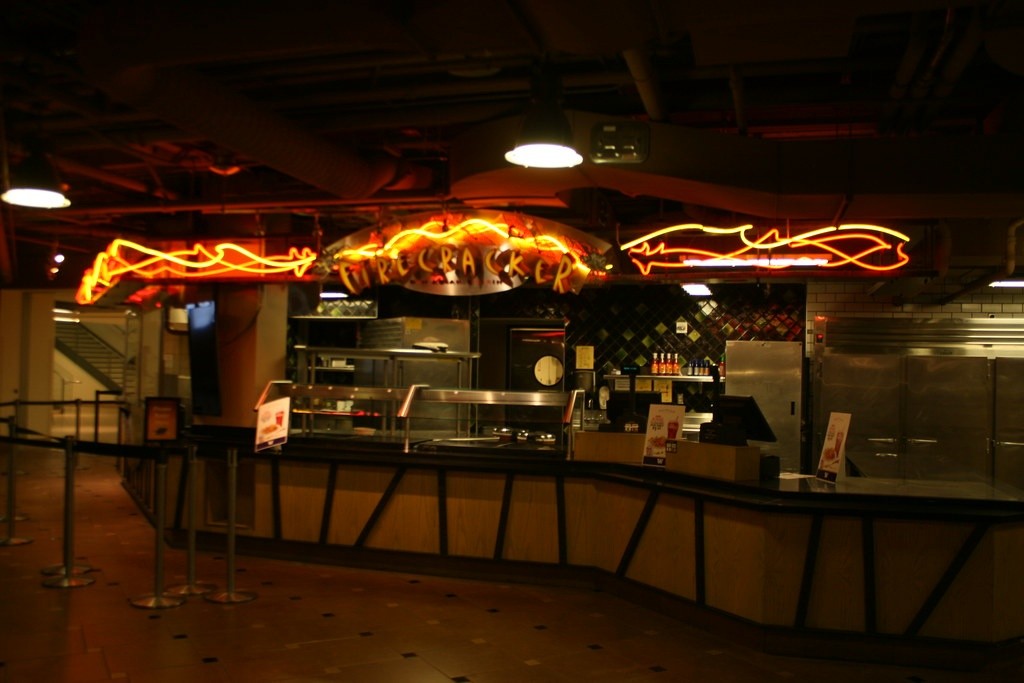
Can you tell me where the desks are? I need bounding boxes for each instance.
[604,374,714,394]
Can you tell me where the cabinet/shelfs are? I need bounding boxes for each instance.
[725,340,802,474]
[811,313,1024,501]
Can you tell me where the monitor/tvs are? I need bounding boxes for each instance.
[606,390,662,432]
[718,395,777,443]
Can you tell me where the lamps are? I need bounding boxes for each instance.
[1,136,71,210]
[319,282,348,299]
[504,58,584,171]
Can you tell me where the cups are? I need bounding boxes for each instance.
[835,433,844,455]
[668,420,678,439]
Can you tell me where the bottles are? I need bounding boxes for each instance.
[719,356,725,377]
[688,360,710,376]
[673,353,680,376]
[659,353,665,376]
[651,353,658,376]
[665,353,673,375]
